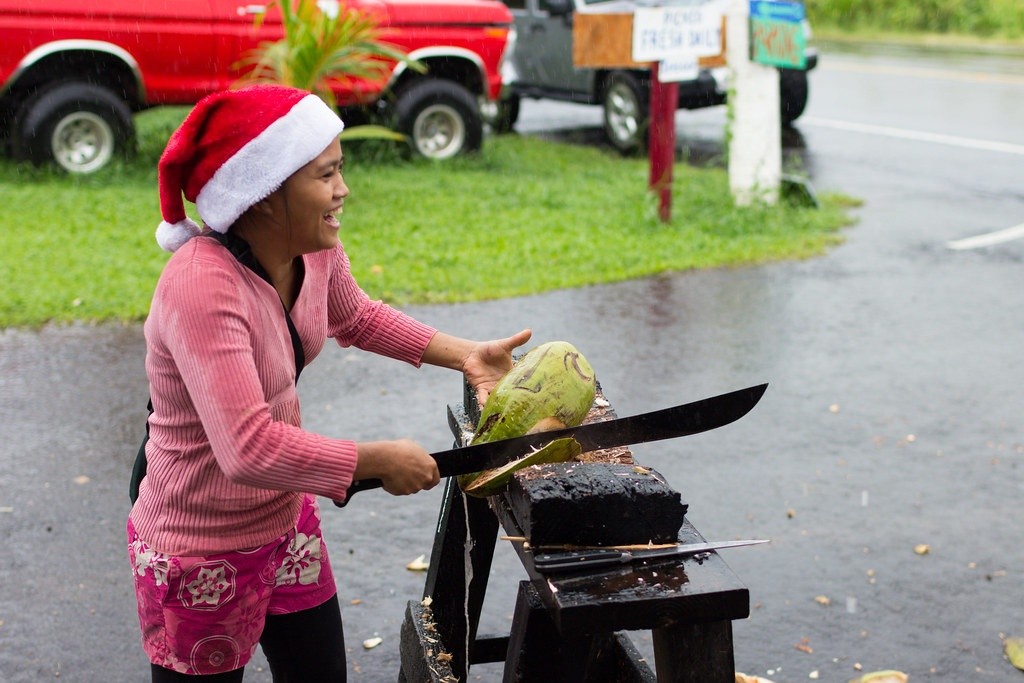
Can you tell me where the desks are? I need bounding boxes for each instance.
[396,401,749,683]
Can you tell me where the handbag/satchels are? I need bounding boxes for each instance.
[129,398,153,505]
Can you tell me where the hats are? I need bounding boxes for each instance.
[161,86,344,249]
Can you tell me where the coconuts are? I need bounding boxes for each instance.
[453,339,597,498]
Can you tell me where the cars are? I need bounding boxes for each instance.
[489,0,822,155]
[0,0,512,173]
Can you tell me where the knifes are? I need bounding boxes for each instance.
[332,383,770,509]
[534,540,770,573]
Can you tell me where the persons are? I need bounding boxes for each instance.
[127,86,532,683]
